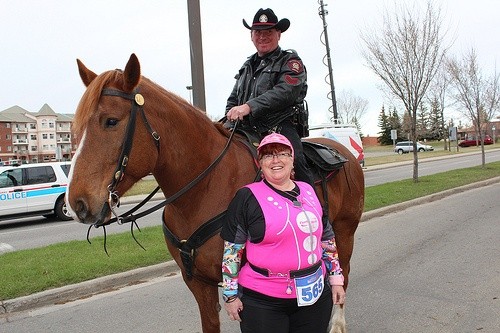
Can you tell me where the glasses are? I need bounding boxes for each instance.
[260,153,292,160]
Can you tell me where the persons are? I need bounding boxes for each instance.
[223,8,323,210]
[220,133,346,333]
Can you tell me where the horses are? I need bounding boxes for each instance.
[62,54,365,333]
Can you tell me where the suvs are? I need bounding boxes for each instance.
[394,141,434,154]
[0,160,74,221]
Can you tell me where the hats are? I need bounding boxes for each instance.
[256,133,294,160]
[243,8,290,33]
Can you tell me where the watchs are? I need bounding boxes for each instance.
[223,295,238,303]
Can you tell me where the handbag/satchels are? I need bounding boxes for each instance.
[301,139,349,172]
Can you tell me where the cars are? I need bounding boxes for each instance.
[459,135,494,146]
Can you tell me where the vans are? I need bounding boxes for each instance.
[301,123,365,167]
